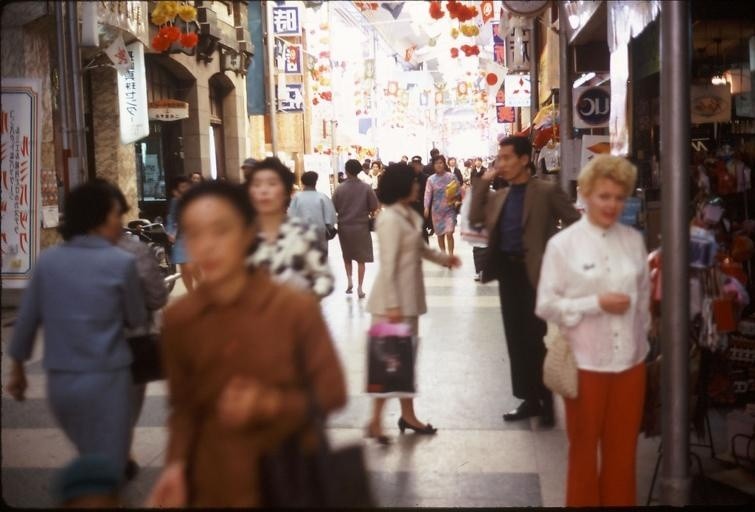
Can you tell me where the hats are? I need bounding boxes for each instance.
[240,158,256,169]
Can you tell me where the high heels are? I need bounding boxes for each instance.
[397,419,436,435]
[364,422,395,445]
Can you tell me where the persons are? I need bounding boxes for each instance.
[166,148,380,300]
[534,154,652,507]
[4,180,148,509]
[119,196,168,481]
[365,163,461,446]
[467,135,581,428]
[355,149,510,281]
[143,179,348,508]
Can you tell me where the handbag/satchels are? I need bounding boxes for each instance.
[326,226,337,241]
[258,445,377,510]
[367,218,375,232]
[127,329,168,386]
[543,319,578,398]
[366,322,417,398]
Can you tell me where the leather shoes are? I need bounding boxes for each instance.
[539,400,555,431]
[503,401,541,421]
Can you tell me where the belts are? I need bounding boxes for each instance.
[502,255,523,264]
